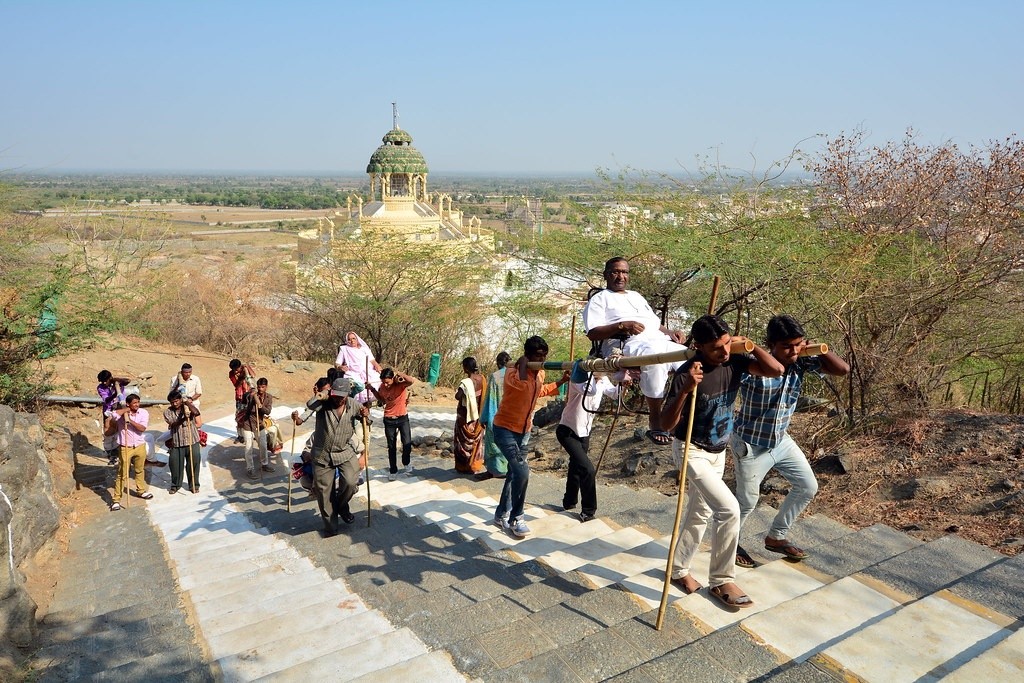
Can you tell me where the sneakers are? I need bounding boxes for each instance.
[248,469,260,480]
[389,472,398,481]
[261,464,275,472]
[494,512,511,530]
[579,511,596,522]
[509,513,530,536]
[404,462,412,473]
[563,502,577,511]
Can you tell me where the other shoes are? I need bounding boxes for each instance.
[191,488,199,493]
[168,487,179,494]
[377,400,385,407]
[339,509,355,524]
[106,448,121,465]
[305,493,315,502]
[363,401,371,408]
[151,460,167,467]
[233,436,246,445]
[324,528,338,539]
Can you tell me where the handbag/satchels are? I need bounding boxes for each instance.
[292,462,305,479]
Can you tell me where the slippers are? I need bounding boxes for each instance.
[735,550,756,567]
[645,428,675,445]
[764,542,810,560]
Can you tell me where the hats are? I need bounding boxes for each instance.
[330,378,352,398]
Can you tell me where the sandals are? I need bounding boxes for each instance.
[708,584,754,608]
[110,502,120,512]
[665,572,704,595]
[137,491,153,499]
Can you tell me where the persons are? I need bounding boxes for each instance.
[731,315,850,570]
[557,341,629,523]
[454,356,486,474]
[335,331,382,403]
[307,379,368,535]
[243,377,276,480]
[301,427,367,493]
[323,367,364,399]
[96,369,128,467]
[227,359,257,445]
[365,368,415,481]
[493,336,572,536]
[163,391,202,494]
[170,363,202,414]
[582,256,690,445]
[480,351,513,478]
[662,315,785,608]
[110,394,153,511]
[291,378,369,443]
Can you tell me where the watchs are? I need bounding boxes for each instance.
[618,321,624,334]
